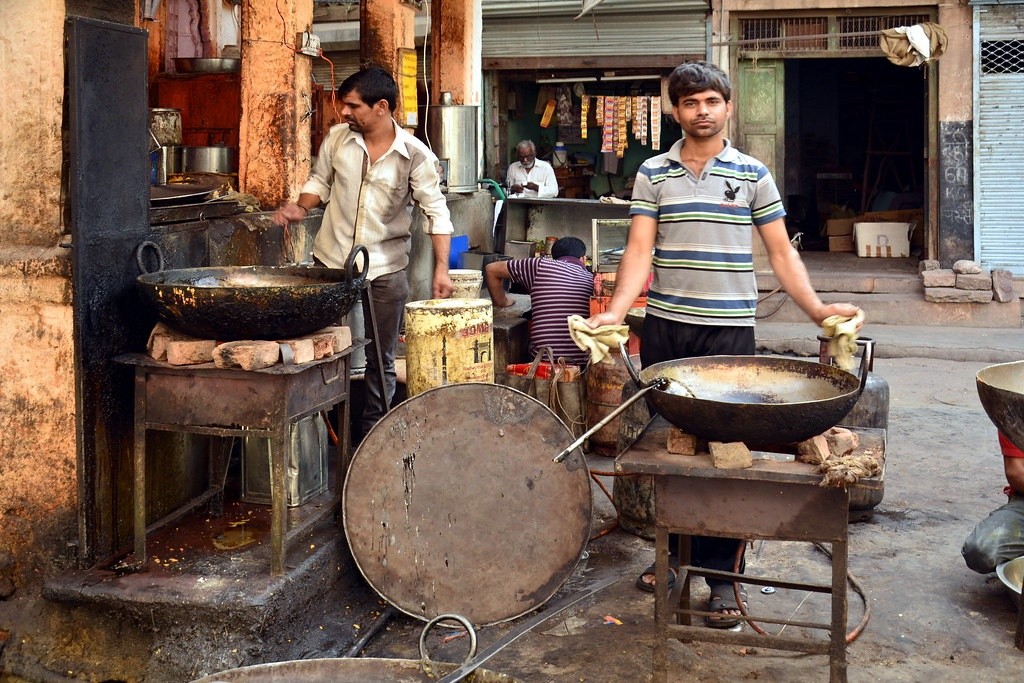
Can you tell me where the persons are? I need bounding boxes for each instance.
[276,67,455,454]
[506,140,559,201]
[582,59,865,628]
[484,236,595,375]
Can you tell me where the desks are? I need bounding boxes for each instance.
[605,411,887,683]
[110,328,370,582]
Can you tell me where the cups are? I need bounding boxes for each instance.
[448,268,483,299]
[169,57,241,74]
[995,555,1024,607]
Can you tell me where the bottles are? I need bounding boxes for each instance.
[544,237,558,255]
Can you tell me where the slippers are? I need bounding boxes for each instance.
[636,557,681,593]
[706,583,749,628]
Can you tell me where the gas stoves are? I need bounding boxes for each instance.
[615,413,887,683]
[113,337,372,576]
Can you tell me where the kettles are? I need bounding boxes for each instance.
[552,142,567,166]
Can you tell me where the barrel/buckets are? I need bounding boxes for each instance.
[404,297,495,401]
[419,92,478,193]
[241,410,330,509]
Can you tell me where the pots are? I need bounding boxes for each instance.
[617,339,873,447]
[146,105,240,195]
[975,357,1024,452]
[134,238,369,338]
